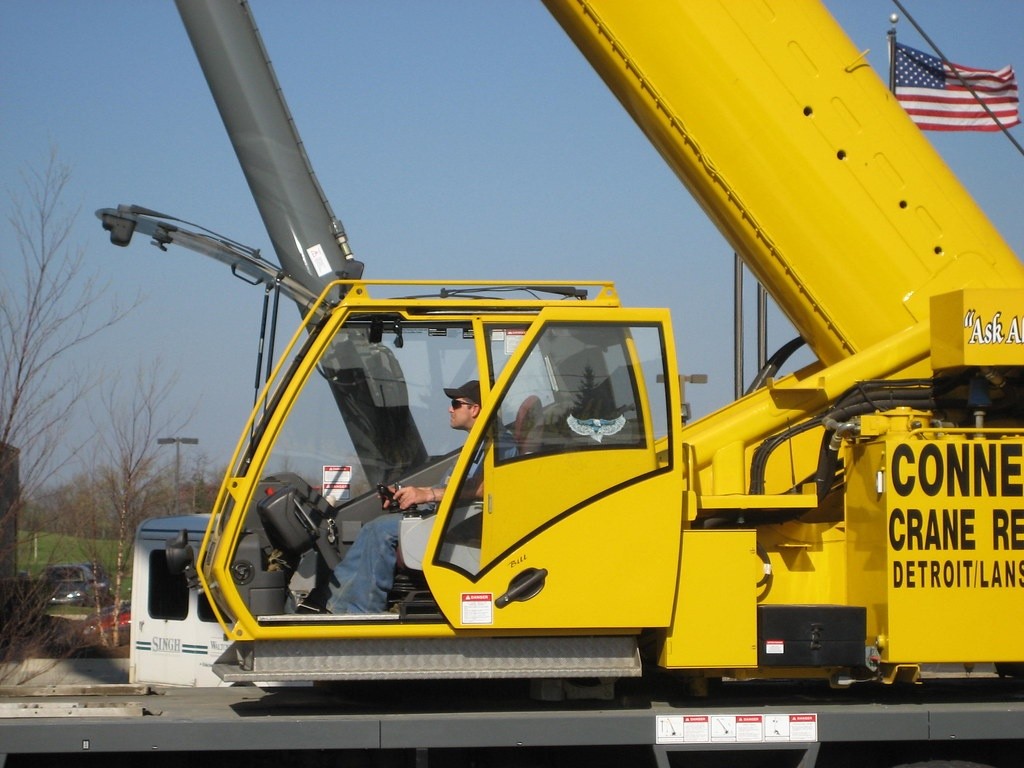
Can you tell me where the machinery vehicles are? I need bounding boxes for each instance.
[0,0,1024,768]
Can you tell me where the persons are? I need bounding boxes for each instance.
[321,378,519,616]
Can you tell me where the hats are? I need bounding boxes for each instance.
[443,380,481,407]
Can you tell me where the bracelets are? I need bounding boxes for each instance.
[430,489,436,501]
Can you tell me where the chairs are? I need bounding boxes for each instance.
[513,394,544,456]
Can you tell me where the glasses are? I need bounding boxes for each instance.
[451,399,474,410]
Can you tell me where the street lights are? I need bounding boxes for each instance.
[157,437,200,517]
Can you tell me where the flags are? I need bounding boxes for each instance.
[894,42,1021,131]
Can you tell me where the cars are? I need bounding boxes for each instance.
[16,562,112,607]
